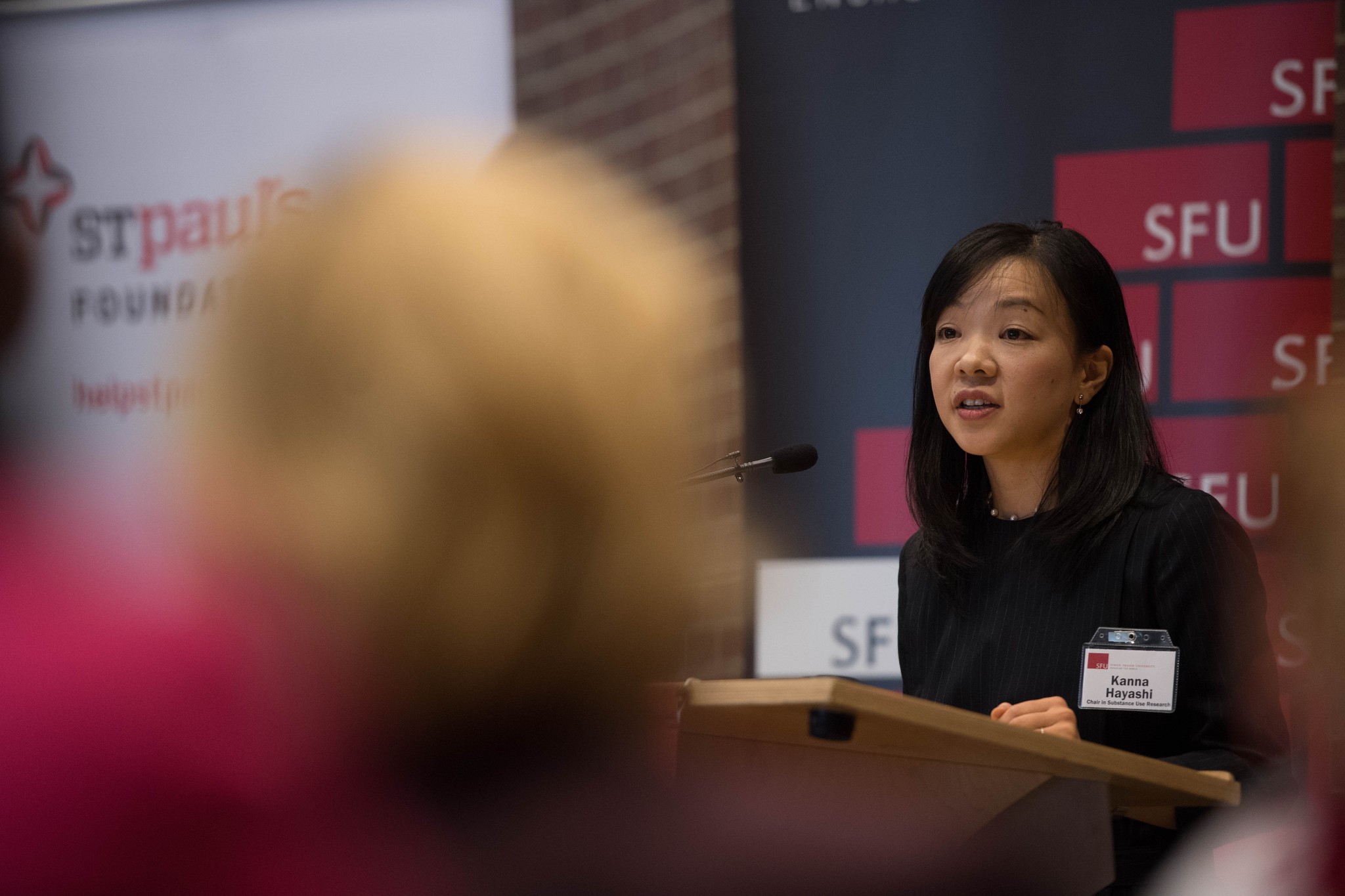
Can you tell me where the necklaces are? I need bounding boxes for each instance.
[987,491,1057,521]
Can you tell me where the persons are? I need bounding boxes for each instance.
[0,134,1344,896]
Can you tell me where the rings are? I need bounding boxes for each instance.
[1041,728,1044,734]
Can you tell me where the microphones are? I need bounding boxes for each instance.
[680,444,818,487]
